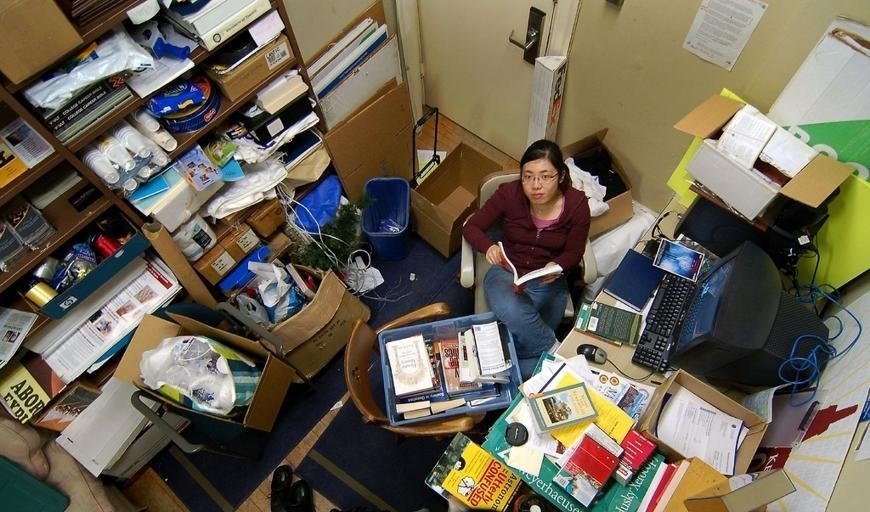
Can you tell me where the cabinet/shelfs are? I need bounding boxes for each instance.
[1,0,331,422]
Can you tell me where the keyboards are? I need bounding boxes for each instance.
[632,272,696,375]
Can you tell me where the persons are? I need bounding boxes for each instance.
[462,140,590,380]
[444,446,466,471]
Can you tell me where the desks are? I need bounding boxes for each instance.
[446,196,828,512]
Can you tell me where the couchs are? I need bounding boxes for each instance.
[0,418,142,512]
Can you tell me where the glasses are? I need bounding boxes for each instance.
[521,172,558,184]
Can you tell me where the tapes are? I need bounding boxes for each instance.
[24,281,58,308]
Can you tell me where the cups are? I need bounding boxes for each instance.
[71,110,178,191]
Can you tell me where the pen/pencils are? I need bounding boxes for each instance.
[651,380,661,385]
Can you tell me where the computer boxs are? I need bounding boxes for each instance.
[673,191,811,269]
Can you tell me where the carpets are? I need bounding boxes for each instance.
[147,233,460,512]
[294,269,576,511]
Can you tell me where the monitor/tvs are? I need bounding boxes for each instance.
[668,239,830,393]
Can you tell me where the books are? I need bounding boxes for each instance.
[574,302,623,347]
[385,320,512,419]
[527,382,599,434]
[547,372,635,449]
[179,0,224,24]
[610,428,657,487]
[252,69,309,115]
[424,432,522,512]
[584,301,643,347]
[555,422,625,470]
[498,241,564,294]
[553,434,619,508]
[286,262,315,300]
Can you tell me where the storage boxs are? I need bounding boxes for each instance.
[207,33,296,102]
[667,94,857,225]
[31,378,102,432]
[561,127,634,238]
[230,260,371,381]
[54,380,191,480]
[637,369,769,479]
[113,311,297,432]
[410,142,504,260]
[191,198,287,287]
[14,208,150,319]
[0,0,84,85]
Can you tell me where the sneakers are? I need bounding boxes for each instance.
[291,479,315,511]
[270,466,294,512]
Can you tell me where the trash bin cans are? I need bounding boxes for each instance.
[361,178,411,261]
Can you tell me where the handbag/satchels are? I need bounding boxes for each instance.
[139,335,261,418]
[249,261,305,324]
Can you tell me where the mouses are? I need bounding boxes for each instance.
[577,344,607,365]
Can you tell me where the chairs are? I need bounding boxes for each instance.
[131,303,318,460]
[461,170,597,323]
[345,302,488,440]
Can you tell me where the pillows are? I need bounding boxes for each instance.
[0,455,68,512]
